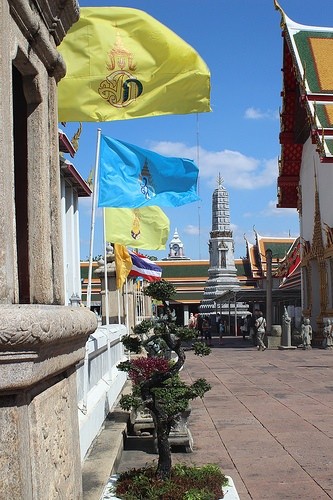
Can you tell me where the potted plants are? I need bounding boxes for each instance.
[98,279,239,500]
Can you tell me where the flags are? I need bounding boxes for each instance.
[97,133,202,209]
[57,6,210,123]
[104,205,170,249]
[112,243,163,289]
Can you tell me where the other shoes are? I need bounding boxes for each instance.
[262,347,266,351]
[258,348,260,350]
[209,344,214,346]
[219,342,223,344]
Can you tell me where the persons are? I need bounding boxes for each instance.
[77,299,299,351]
[323,320,333,350]
[299,318,312,350]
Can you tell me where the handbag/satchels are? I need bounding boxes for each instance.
[254,329,258,334]
